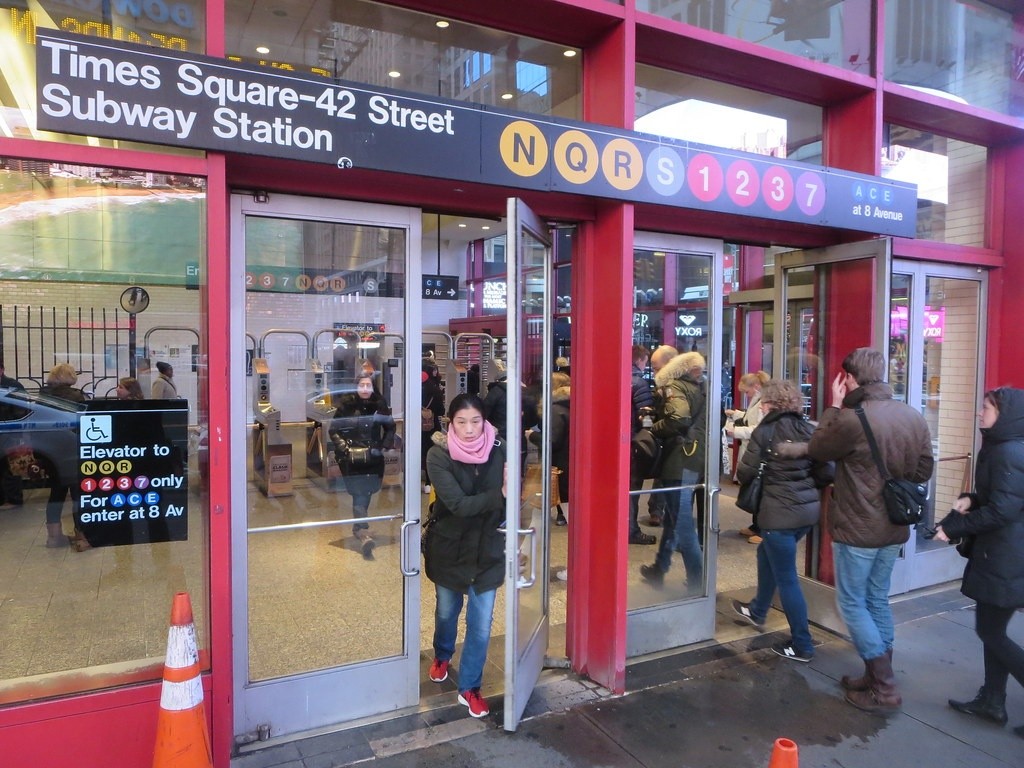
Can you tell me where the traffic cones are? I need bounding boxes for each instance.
[151,590,216,768]
[767,737,802,768]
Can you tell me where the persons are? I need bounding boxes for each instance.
[425,394,505,717]
[725,370,770,544]
[116,377,144,399]
[152,361,178,398]
[328,373,395,554]
[809,346,933,711]
[0,362,30,510]
[632,344,726,598]
[933,386,1024,737]
[421,357,445,493]
[30,364,93,551]
[474,373,570,526]
[731,379,835,659]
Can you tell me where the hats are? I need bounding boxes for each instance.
[156,362,172,374]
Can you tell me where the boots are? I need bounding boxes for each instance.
[1014,725,1024,738]
[844,649,893,691]
[355,528,376,557]
[73,528,93,552]
[845,655,902,714]
[45,521,76,547]
[948,684,1008,726]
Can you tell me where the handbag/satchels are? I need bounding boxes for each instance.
[422,407,435,431]
[733,418,745,446]
[421,502,440,553]
[735,472,762,514]
[346,445,371,469]
[883,479,928,530]
[922,508,976,558]
[812,459,836,490]
[631,429,664,478]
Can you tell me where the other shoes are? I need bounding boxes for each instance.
[557,569,567,581]
[556,505,565,525]
[649,514,660,525]
[747,534,763,544]
[631,531,656,544]
[740,528,755,535]
[640,564,663,585]
[422,481,431,494]
[0,503,21,510]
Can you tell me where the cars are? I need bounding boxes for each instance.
[0,386,92,493]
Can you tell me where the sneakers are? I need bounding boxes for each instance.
[731,599,766,627]
[771,639,814,662]
[429,657,452,682]
[458,689,489,717]
